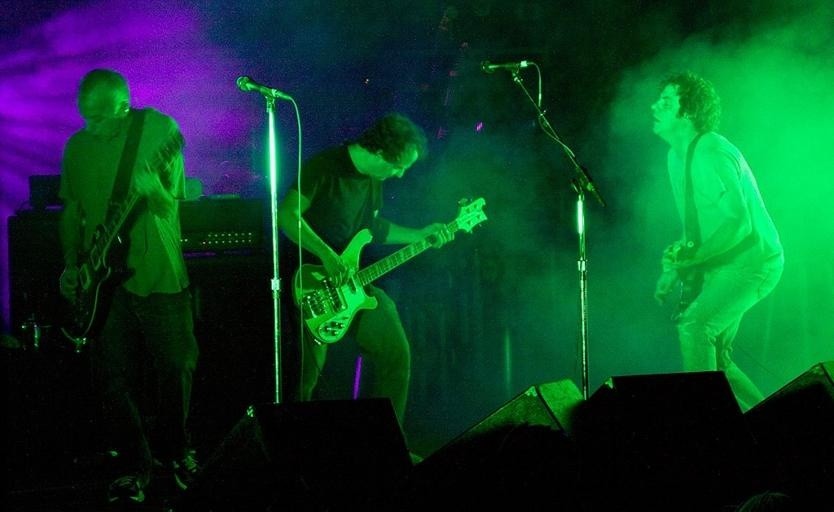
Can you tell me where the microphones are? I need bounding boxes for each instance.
[480,59,534,74]
[236,76,292,102]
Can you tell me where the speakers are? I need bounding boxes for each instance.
[744,362,834,489]
[573,371,744,489]
[418,379,584,463]
[173,398,415,512]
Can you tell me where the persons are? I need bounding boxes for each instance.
[275,109,456,465]
[208,421,834,512]
[650,73,785,414]
[57,70,202,506]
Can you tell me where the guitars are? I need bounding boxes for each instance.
[293,197,488,345]
[57,129,184,340]
[663,239,703,314]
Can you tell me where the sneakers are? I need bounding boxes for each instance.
[151,451,198,489]
[108,474,148,503]
